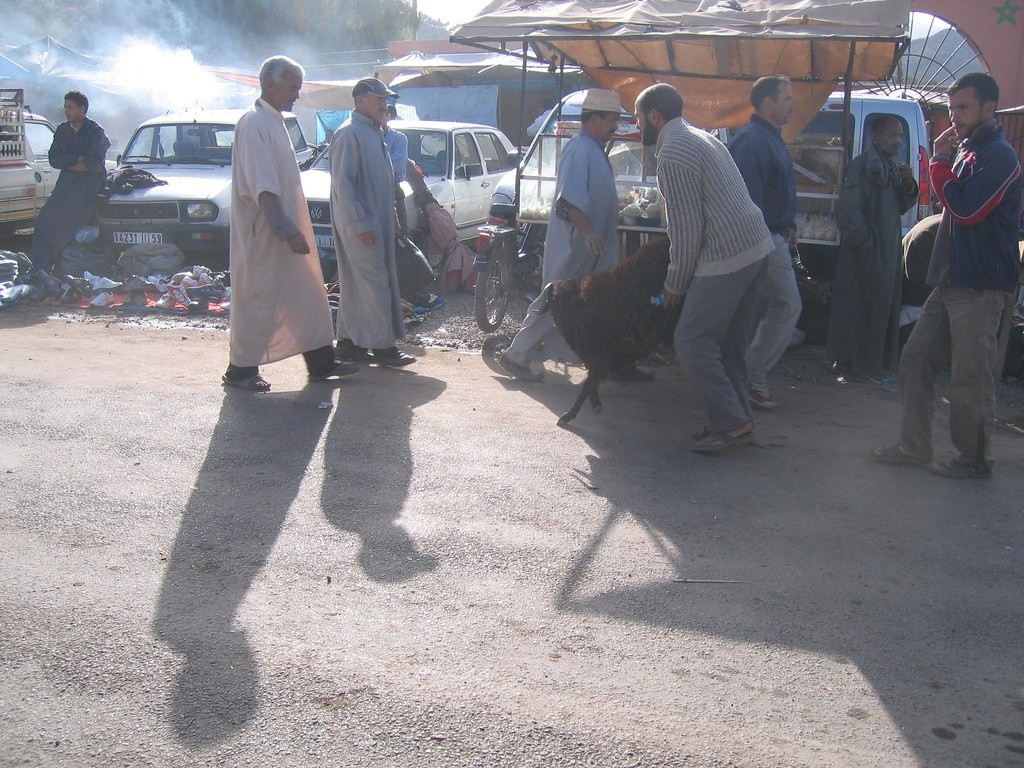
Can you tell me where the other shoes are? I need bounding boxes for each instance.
[837,360,883,383]
[499,352,544,382]
[929,458,992,479]
[611,366,656,381]
[335,340,374,362]
[373,346,416,367]
[871,445,932,466]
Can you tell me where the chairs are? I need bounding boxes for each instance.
[172,142,192,158]
[438,150,459,168]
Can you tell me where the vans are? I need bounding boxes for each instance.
[485,90,933,293]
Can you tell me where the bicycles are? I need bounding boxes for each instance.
[473,185,544,335]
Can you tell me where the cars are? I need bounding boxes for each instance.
[93,107,314,255]
[299,120,528,262]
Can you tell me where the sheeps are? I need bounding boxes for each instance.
[533,239,678,426]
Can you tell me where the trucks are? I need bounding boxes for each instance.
[0,111,127,240]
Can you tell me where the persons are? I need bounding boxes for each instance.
[727,74,800,411]
[827,117,919,383]
[16,89,111,300]
[870,72,1021,477]
[329,76,417,367]
[496,89,657,383]
[222,54,359,391]
[379,105,408,318]
[634,83,776,453]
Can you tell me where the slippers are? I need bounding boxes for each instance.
[691,423,756,451]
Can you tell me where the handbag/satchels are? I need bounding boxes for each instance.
[395,231,435,300]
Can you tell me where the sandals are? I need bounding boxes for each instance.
[308,364,359,380]
[222,373,270,391]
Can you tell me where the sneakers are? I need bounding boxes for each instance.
[748,381,779,410]
[1,265,231,313]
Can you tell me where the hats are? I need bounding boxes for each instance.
[569,89,629,114]
[352,78,396,97]
